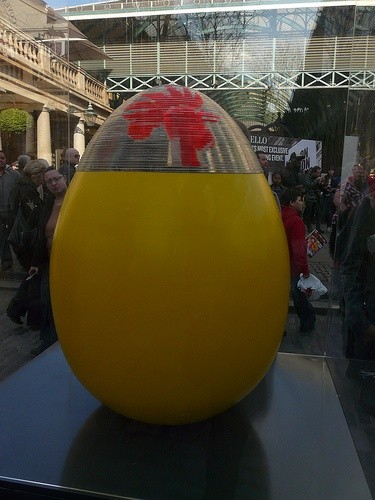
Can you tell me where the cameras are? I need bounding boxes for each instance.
[26,272,41,280]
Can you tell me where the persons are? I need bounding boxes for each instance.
[254,150,375,384]
[0,148,80,342]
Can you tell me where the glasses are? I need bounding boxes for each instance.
[45,176,62,185]
[71,155,80,158]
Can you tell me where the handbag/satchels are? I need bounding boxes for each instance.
[8,207,29,246]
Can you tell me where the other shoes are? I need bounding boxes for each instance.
[7,312,22,325]
[30,338,57,356]
[316,227,325,234]
